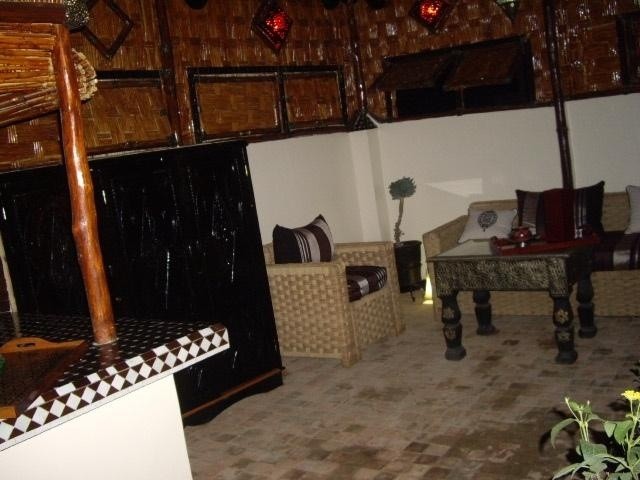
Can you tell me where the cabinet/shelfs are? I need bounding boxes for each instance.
[0,138,285,428]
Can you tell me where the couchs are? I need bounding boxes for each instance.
[422,192,640,316]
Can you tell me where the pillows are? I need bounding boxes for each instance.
[543,181,605,243]
[458,208,518,244]
[272,214,334,264]
[515,190,544,239]
[624,185,640,234]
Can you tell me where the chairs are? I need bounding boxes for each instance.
[262,241,405,369]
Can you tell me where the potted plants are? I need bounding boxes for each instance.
[389,177,426,302]
